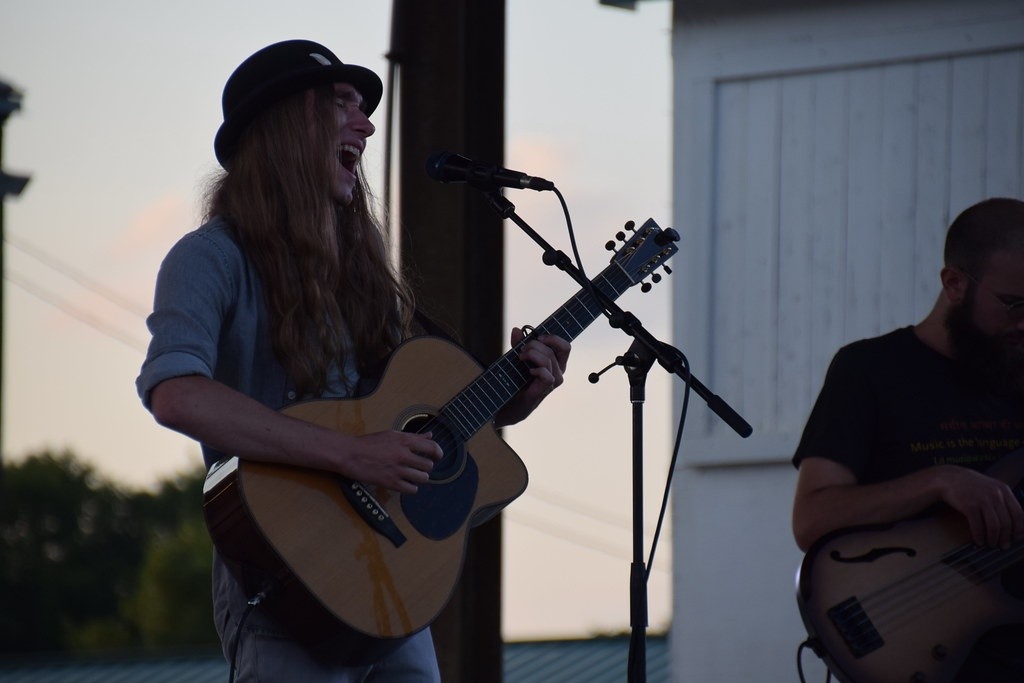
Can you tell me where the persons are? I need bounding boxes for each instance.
[134,39,570,683]
[792,196,1024,683]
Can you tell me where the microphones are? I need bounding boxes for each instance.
[420,150,554,193]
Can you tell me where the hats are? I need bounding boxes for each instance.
[213,40,386,170]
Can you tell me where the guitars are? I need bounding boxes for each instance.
[795,499,1024,683]
[202,216,679,670]
